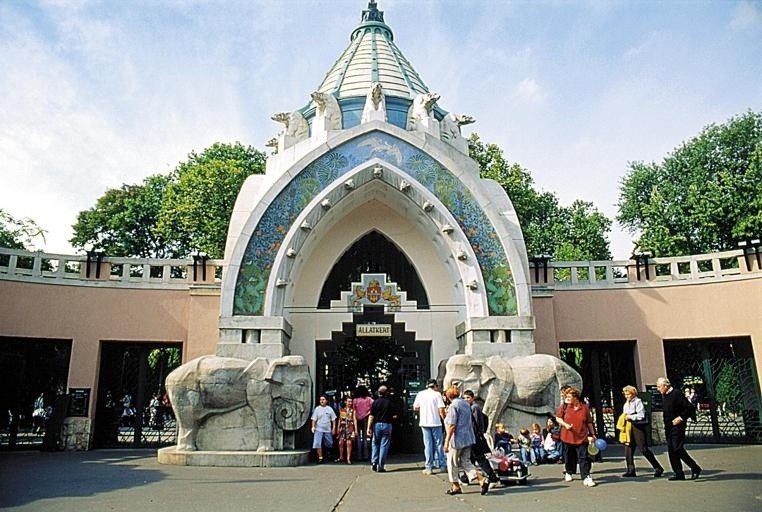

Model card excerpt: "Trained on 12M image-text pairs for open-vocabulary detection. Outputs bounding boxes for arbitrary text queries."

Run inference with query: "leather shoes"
[372,463,377,472]
[691,464,702,480]
[622,472,635,477]
[654,468,664,478]
[378,469,386,472]
[668,472,685,481]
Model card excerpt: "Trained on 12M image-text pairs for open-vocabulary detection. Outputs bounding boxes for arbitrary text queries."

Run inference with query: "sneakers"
[582,475,596,487]
[564,472,573,482]
[422,468,432,474]
[315,458,324,464]
[488,480,503,489]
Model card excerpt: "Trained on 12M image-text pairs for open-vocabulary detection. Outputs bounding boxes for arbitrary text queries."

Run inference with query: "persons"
[352,386,375,462]
[601,397,609,408]
[366,386,398,472]
[529,423,547,465]
[518,429,538,467]
[386,387,406,458]
[560,386,571,404]
[458,390,501,489]
[441,391,451,419]
[0,391,176,436]
[328,393,339,411]
[413,379,448,475]
[544,432,560,463]
[334,397,358,465]
[583,397,592,412]
[443,385,490,495]
[616,385,664,478]
[542,417,563,465]
[310,395,337,465]
[717,400,739,417]
[556,388,596,487]
[494,423,518,454]
[684,387,699,413]
[656,377,702,481]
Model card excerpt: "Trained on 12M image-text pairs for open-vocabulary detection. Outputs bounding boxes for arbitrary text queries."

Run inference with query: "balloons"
[587,445,599,455]
[595,439,606,449]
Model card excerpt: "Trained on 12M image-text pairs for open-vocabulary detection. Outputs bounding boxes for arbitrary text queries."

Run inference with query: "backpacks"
[472,409,488,434]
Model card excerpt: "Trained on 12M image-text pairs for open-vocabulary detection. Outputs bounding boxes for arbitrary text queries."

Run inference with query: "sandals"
[444,487,463,494]
[346,459,351,465]
[334,459,344,464]
[481,477,491,495]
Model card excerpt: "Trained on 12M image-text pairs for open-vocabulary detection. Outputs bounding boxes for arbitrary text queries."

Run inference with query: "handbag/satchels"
[631,398,651,428]
